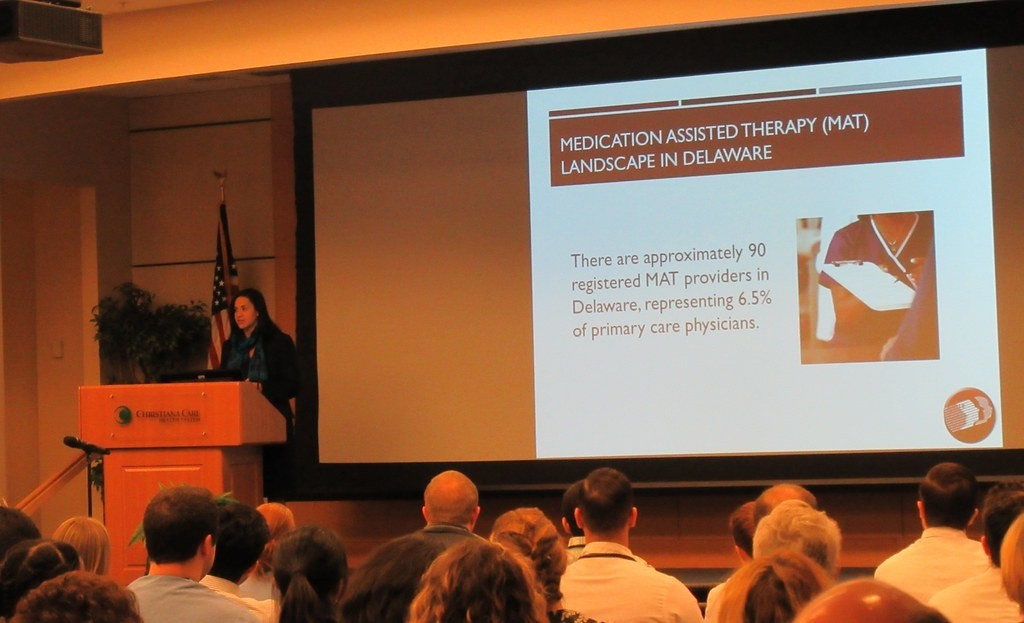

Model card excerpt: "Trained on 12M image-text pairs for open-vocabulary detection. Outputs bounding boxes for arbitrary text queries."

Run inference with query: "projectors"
[0,0,104,64]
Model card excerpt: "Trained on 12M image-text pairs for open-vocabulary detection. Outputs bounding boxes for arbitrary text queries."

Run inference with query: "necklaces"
[880,213,912,252]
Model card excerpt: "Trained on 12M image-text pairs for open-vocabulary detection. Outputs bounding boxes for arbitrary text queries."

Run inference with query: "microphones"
[64,436,110,455]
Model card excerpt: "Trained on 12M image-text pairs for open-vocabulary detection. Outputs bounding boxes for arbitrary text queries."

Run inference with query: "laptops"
[159,368,246,382]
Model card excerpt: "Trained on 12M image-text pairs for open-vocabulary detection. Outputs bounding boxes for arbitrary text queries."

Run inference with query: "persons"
[0,463,1024,623]
[818,211,939,361]
[199,287,300,503]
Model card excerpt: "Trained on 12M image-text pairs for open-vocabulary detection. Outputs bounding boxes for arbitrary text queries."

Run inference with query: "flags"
[206,201,241,370]
